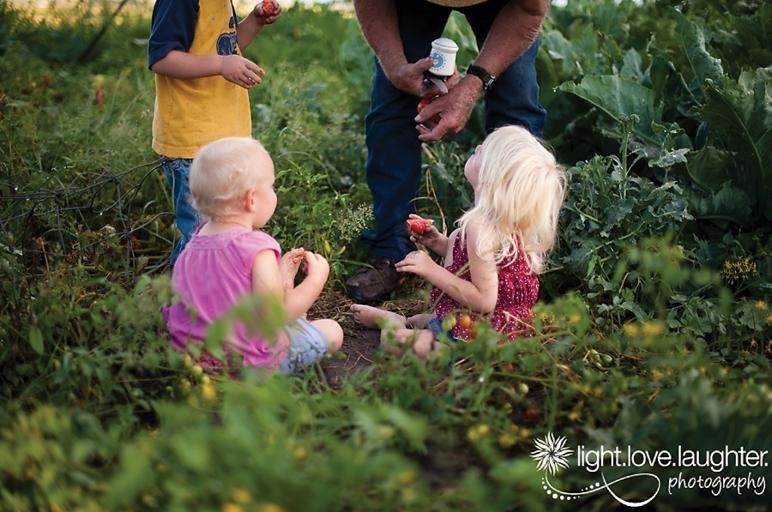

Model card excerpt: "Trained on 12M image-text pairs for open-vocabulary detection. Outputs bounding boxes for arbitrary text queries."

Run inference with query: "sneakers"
[346,249,416,305]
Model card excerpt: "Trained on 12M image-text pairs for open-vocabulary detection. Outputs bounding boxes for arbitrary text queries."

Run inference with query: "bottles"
[427,36,461,77]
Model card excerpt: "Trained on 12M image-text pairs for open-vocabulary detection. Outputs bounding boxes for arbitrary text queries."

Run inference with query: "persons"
[345,0,556,305]
[149,0,281,264]
[155,137,346,379]
[350,124,571,359]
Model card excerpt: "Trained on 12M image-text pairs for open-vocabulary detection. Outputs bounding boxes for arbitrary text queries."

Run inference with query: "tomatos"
[417,98,433,120]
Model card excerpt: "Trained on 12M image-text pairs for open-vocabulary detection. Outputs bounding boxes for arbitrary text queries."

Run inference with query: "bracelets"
[468,63,495,93]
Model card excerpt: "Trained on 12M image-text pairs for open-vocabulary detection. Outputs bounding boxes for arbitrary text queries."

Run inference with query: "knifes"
[430,78,449,94]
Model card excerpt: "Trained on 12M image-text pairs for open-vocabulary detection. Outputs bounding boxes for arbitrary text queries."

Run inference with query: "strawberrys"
[262,0,275,17]
[411,221,425,235]
